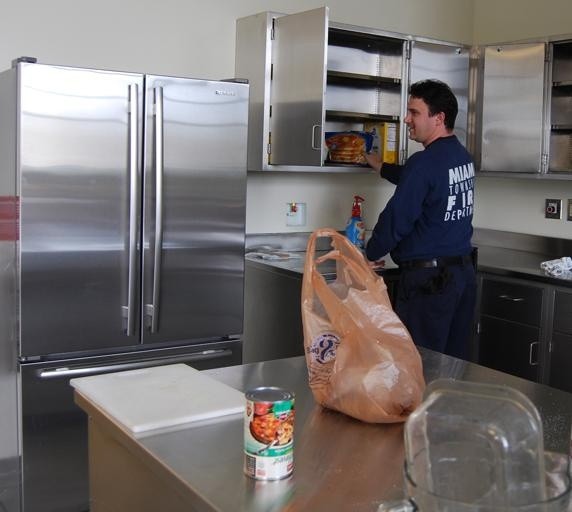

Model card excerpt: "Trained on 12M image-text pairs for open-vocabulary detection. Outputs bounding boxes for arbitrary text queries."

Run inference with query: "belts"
[396,254,474,269]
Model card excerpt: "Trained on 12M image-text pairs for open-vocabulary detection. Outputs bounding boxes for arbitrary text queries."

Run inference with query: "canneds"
[242,387,298,482]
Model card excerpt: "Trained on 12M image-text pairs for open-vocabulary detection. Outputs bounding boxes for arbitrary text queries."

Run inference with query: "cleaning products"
[345,195,366,248]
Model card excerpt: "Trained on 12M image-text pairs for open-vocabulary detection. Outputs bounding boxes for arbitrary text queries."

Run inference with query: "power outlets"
[545,199,561,220]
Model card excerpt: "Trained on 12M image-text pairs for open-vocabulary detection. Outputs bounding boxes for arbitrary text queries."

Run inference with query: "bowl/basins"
[401,457,570,511]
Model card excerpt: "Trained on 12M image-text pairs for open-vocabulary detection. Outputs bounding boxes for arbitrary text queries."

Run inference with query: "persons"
[361,79,477,359]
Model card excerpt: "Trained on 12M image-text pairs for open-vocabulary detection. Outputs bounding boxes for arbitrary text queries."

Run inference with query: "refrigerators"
[0,57,250,512]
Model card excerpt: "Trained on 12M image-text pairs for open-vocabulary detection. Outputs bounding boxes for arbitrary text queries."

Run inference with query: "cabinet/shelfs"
[545,287,572,393]
[237,6,408,172]
[406,37,471,167]
[478,275,546,381]
[481,39,572,179]
